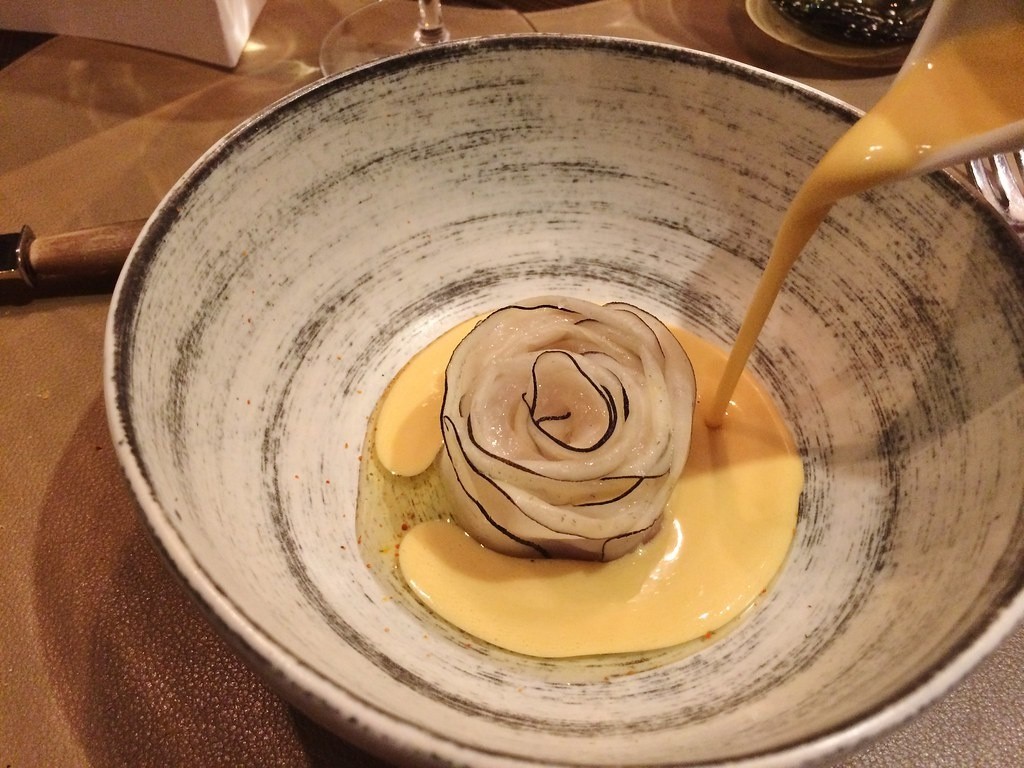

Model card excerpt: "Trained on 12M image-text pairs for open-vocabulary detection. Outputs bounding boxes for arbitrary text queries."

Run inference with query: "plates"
[746,0,921,58]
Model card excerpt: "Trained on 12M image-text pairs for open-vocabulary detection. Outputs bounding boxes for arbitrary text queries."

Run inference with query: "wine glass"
[319,0,536,79]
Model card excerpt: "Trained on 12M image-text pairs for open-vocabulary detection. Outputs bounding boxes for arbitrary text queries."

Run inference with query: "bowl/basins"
[102,33,1024,767]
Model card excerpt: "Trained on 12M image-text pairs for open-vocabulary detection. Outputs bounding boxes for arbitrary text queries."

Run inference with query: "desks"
[0,0,1024,768]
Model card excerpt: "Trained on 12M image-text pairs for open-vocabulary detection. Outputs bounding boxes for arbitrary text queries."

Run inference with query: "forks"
[966,148,1024,227]
